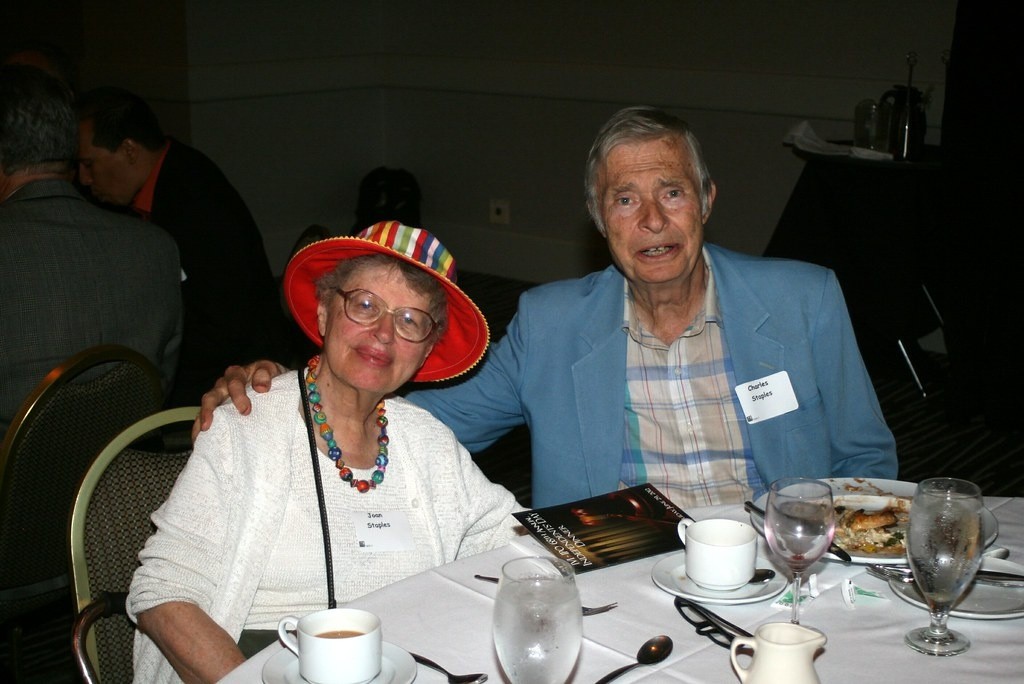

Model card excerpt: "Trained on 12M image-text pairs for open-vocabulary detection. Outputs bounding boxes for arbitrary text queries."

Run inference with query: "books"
[512,483,696,579]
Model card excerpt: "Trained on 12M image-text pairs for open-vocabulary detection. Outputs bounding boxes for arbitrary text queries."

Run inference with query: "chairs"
[0,346,165,684]
[71,407,201,684]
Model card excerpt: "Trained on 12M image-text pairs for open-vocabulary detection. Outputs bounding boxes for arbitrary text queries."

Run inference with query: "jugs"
[730,622,827,683]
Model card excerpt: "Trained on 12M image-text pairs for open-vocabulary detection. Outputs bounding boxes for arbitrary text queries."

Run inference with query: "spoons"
[594,635,674,684]
[748,569,776,584]
[410,652,488,684]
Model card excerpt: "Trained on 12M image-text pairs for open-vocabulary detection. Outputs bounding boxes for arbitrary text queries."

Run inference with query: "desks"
[216,498,1024,684]
[762,142,945,402]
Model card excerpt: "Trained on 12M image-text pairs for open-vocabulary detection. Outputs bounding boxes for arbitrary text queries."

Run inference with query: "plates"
[750,477,999,564]
[652,552,787,605]
[889,555,1014,619]
[261,642,417,684]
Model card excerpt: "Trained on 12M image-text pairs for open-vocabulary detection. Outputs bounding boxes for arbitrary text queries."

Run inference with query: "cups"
[492,556,584,684]
[277,607,381,684]
[678,518,758,591]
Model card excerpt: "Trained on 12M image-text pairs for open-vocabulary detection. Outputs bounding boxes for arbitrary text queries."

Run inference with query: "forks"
[474,574,617,615]
[868,564,1024,588]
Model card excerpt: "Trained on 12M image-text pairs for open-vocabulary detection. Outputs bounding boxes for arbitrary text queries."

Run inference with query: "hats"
[285,221,490,382]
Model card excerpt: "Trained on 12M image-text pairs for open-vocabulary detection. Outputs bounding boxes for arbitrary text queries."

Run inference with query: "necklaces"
[306,354,390,493]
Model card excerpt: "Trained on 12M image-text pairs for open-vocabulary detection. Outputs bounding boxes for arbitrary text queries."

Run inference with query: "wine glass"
[904,478,986,656]
[763,476,835,640]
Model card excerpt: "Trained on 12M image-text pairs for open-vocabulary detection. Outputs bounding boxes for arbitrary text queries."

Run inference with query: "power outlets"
[489,199,513,225]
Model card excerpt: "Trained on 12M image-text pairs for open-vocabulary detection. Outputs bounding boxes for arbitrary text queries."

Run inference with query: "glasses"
[674,596,754,649]
[333,286,440,342]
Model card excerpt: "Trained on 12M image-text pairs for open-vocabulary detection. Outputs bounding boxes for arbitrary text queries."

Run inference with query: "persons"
[126,219,533,684]
[0,62,185,603]
[192,106,900,508]
[76,85,288,434]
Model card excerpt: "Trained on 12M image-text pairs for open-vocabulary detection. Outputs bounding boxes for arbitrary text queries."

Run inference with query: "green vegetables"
[835,505,904,547]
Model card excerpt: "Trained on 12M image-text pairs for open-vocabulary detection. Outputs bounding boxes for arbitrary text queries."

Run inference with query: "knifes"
[744,500,852,563]
